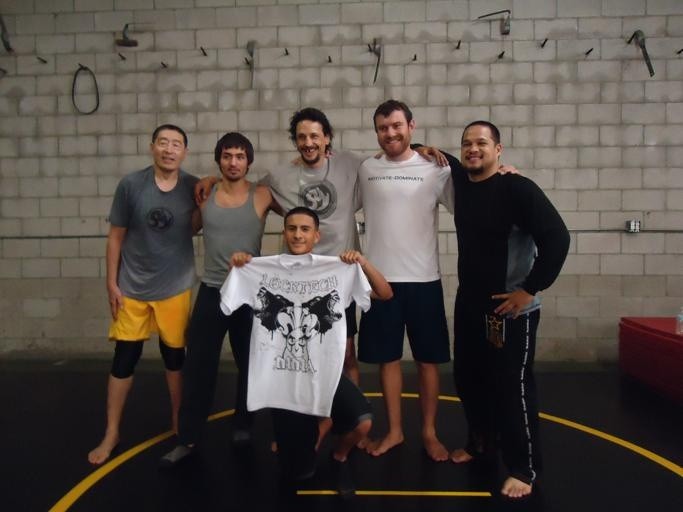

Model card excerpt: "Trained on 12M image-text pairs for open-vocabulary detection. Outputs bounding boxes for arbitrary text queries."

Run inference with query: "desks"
[618,315,683,399]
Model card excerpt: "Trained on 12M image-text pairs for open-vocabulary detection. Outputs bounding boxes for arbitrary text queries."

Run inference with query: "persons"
[193,104,451,455]
[86,122,205,469]
[161,127,275,468]
[342,100,521,463]
[407,118,570,498]
[227,206,392,471]
